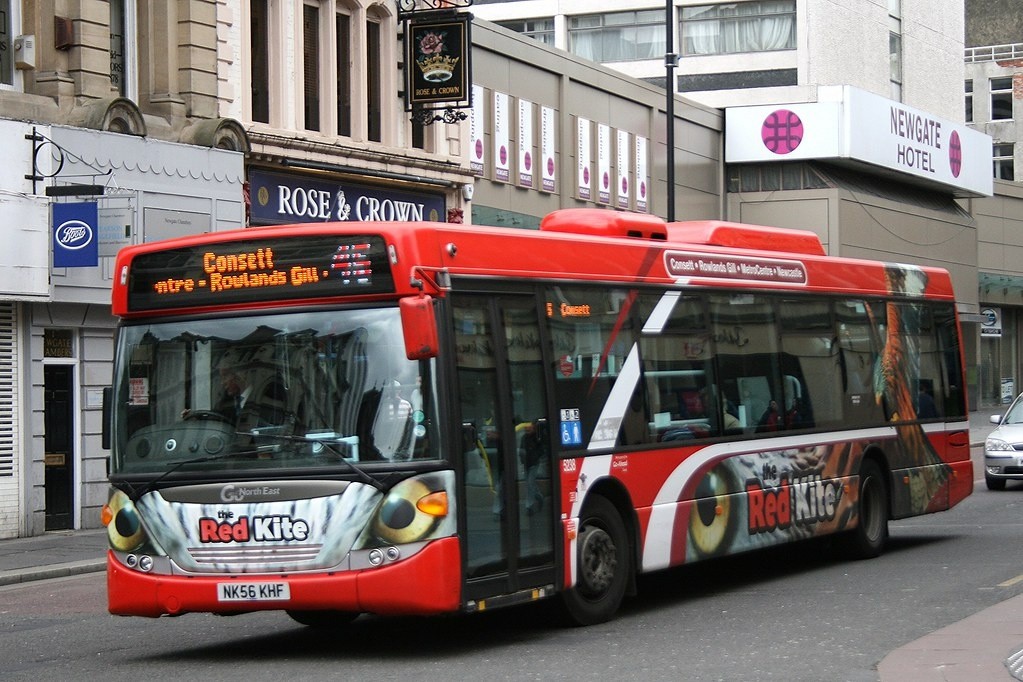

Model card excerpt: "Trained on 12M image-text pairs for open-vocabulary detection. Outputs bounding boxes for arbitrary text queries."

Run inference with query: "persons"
[180,356,268,425]
[737,361,961,433]
[666,368,742,434]
[481,423,544,520]
[381,377,425,419]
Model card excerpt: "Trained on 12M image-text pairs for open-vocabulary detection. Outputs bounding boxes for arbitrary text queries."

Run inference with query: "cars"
[984,391,1023,490]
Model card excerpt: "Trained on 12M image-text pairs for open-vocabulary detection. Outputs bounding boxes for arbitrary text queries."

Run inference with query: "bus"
[98,206,975,631]
